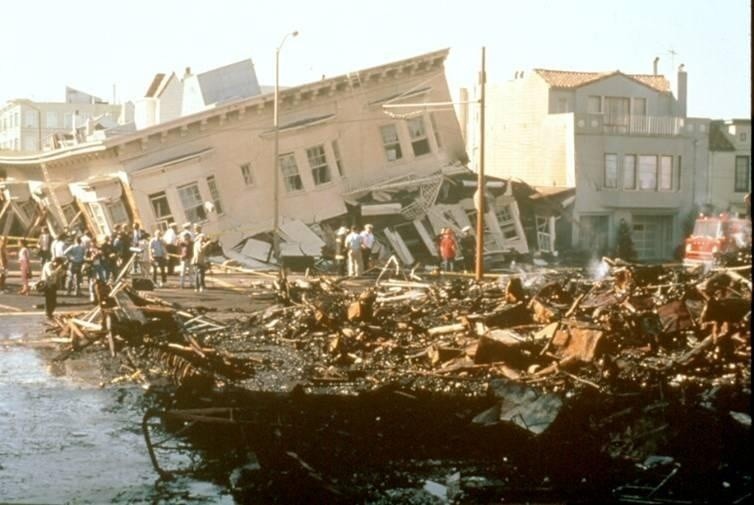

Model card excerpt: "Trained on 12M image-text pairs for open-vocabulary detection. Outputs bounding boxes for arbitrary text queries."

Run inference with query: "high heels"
[18,286,31,297]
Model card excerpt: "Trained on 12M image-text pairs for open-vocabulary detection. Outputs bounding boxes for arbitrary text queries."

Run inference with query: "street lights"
[273,28,300,253]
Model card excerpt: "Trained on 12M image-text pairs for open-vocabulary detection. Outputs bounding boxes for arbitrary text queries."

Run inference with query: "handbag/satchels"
[81,263,90,275]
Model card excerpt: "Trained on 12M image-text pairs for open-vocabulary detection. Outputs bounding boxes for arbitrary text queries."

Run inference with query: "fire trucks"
[681,211,751,268]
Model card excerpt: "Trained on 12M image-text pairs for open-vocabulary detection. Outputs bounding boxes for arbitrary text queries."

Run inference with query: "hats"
[335,224,374,236]
[462,226,470,232]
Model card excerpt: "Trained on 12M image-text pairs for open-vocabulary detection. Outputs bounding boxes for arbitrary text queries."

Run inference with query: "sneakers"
[170,220,203,239]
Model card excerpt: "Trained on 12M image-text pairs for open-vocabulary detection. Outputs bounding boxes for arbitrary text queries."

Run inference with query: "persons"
[433,226,476,274]
[0,236,10,295]
[335,223,374,280]
[17,239,32,295]
[36,221,210,320]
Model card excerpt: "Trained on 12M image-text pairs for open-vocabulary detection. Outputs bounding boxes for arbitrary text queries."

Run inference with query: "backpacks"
[31,281,47,293]
